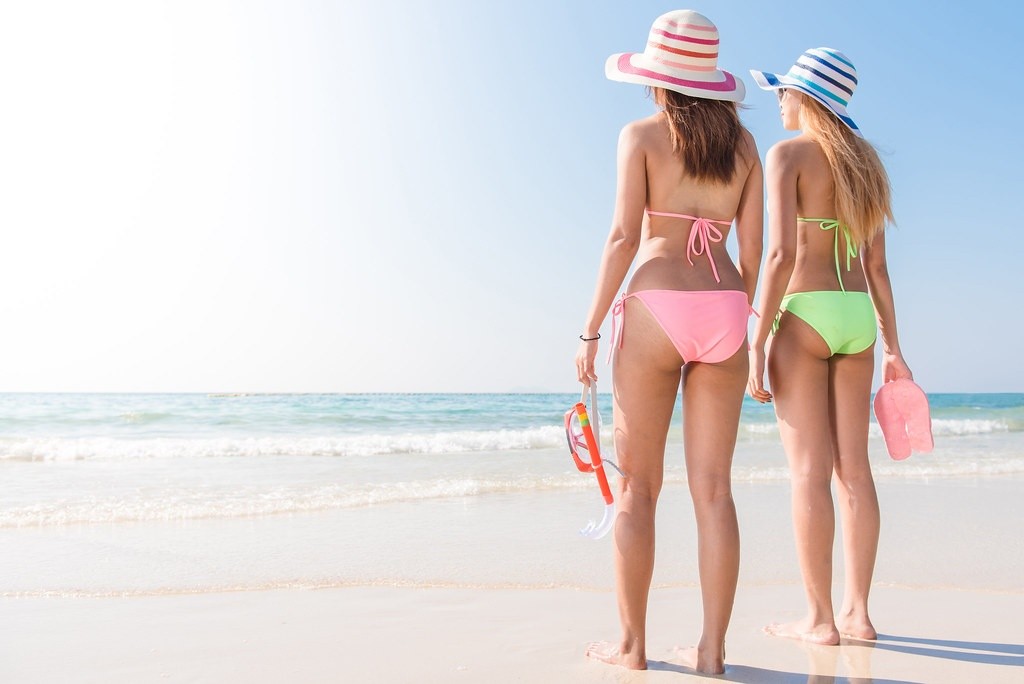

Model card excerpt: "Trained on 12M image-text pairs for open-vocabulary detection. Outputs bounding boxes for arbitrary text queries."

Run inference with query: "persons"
[575,10,916,675]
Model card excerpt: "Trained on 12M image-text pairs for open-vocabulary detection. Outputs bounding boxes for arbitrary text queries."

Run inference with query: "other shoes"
[890,379,935,455]
[873,382,912,461]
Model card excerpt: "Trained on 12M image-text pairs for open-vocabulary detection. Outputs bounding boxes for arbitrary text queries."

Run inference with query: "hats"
[604,10,746,103]
[749,46,865,140]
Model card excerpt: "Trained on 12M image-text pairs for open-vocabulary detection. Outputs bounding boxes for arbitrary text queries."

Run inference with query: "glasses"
[778,88,788,101]
[563,377,601,474]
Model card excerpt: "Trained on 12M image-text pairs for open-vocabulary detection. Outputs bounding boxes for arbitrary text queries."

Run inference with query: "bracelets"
[579,333,601,341]
